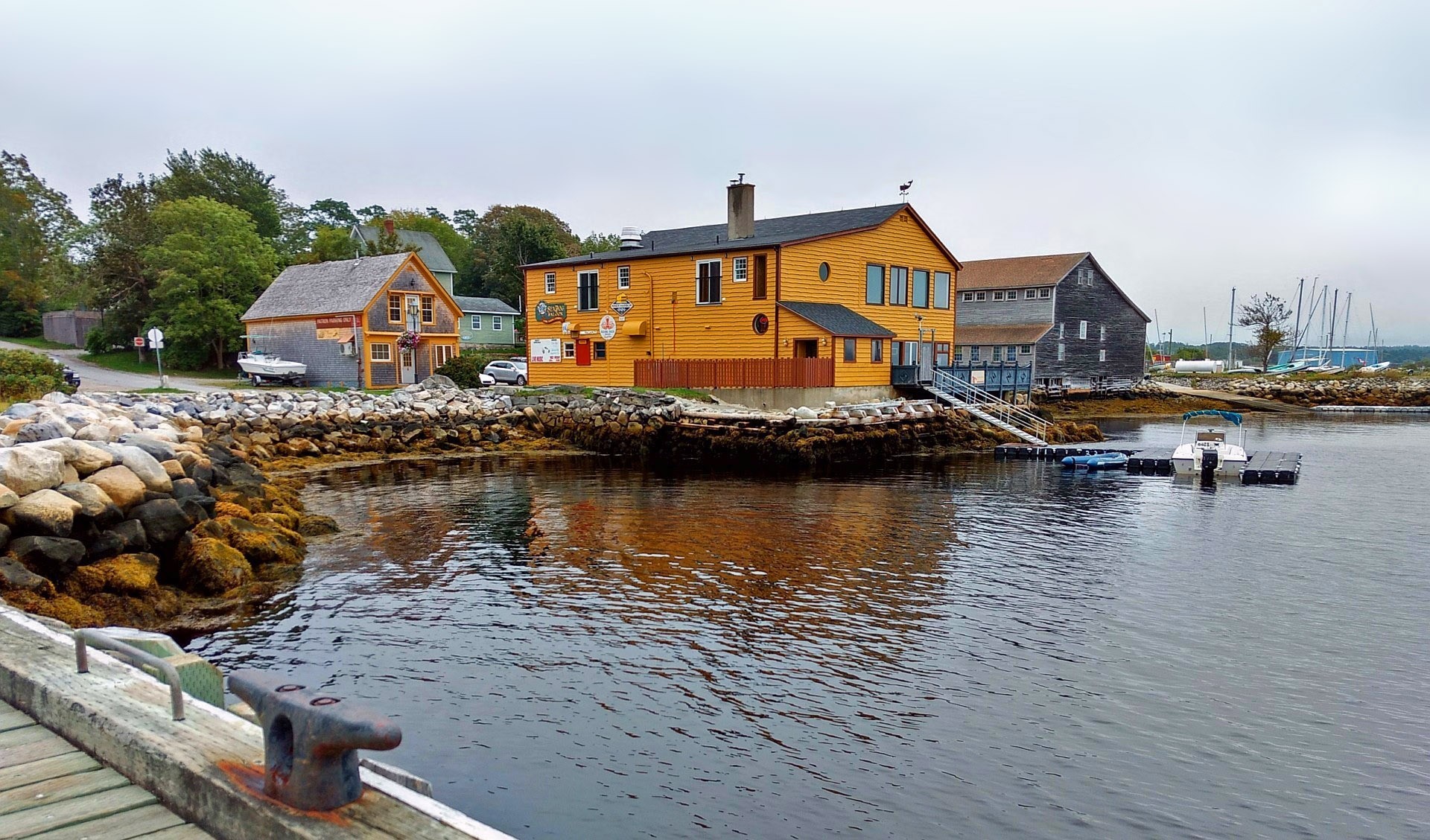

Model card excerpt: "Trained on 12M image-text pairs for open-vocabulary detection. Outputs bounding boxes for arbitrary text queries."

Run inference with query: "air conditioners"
[339,342,352,356]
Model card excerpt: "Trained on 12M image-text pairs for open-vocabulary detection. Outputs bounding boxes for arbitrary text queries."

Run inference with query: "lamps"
[822,336,827,346]
[783,337,788,347]
[411,278,415,285]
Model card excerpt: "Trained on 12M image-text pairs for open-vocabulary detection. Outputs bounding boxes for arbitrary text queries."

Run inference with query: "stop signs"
[134,337,145,347]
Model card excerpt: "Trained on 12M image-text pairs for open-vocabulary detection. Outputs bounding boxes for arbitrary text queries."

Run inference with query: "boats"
[1172,408,1249,477]
[236,335,307,376]
[1062,452,1128,469]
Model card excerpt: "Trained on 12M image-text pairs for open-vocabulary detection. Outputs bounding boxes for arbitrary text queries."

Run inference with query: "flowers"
[395,329,421,355]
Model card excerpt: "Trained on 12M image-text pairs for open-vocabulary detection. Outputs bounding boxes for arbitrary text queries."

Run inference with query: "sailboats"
[1148,309,1166,373]
[1358,301,1390,373]
[1223,275,1351,375]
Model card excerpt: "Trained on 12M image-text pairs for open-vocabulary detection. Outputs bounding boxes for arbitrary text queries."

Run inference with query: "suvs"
[483,360,527,385]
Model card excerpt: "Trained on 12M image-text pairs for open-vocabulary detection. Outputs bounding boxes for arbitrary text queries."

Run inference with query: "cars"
[479,373,494,387]
[61,366,80,388]
